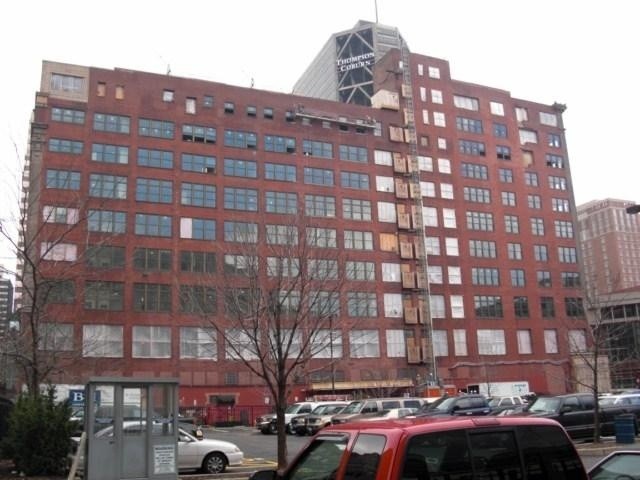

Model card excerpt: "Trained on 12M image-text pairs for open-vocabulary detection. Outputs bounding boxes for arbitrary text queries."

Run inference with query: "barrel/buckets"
[614,414,635,443]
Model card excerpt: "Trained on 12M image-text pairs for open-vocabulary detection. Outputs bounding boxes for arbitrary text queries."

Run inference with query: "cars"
[66,405,244,480]
